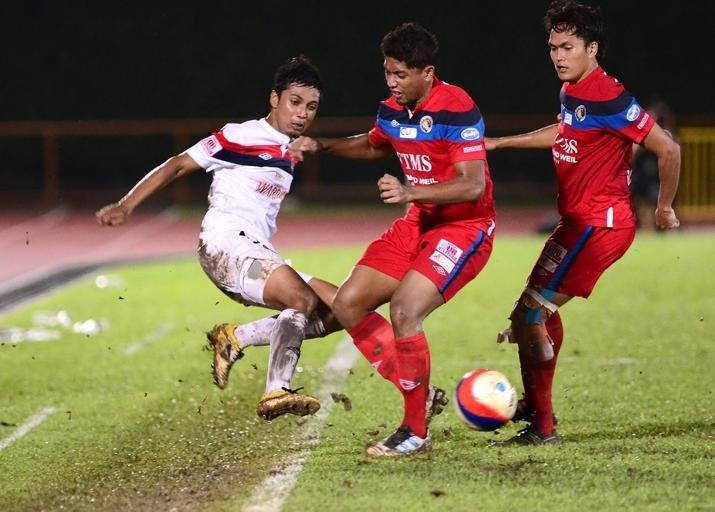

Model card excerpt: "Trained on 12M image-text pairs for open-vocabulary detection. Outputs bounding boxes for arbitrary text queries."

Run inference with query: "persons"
[94,56,345,422]
[287,22,498,457]
[633,101,680,229]
[484,4,682,448]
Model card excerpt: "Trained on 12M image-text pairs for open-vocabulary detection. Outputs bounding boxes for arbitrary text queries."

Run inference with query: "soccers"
[454,369,518,432]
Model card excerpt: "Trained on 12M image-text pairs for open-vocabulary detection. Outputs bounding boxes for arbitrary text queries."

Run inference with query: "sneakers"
[491,426,562,447]
[257,388,321,421]
[212,322,245,391]
[423,383,449,425]
[364,424,431,460]
[511,398,559,426]
